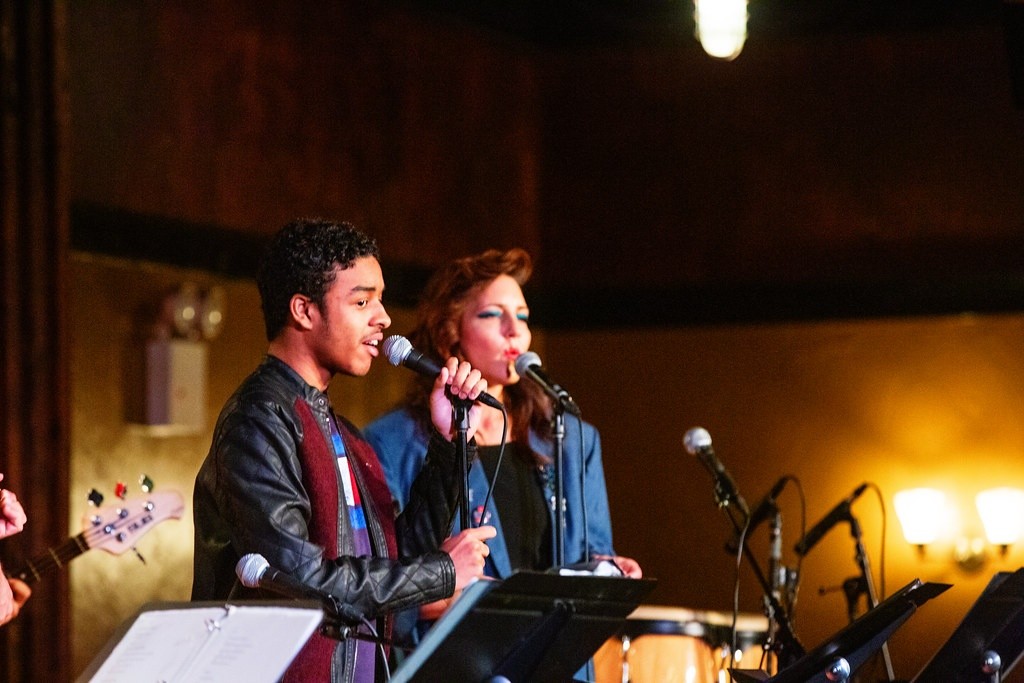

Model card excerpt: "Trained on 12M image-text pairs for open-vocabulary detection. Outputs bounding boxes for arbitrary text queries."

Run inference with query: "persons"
[189,218,498,683]
[0,472,31,627]
[360,248,644,683]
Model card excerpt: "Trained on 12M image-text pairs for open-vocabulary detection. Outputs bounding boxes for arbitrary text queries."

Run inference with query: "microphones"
[724,476,791,555]
[795,483,868,557]
[235,554,365,625]
[513,351,582,417]
[682,426,748,516]
[381,334,505,410]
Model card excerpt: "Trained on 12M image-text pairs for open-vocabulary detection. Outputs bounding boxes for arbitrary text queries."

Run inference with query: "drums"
[591,605,779,683]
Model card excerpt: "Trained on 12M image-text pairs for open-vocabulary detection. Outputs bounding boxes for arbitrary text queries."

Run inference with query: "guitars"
[7,475,185,591]
